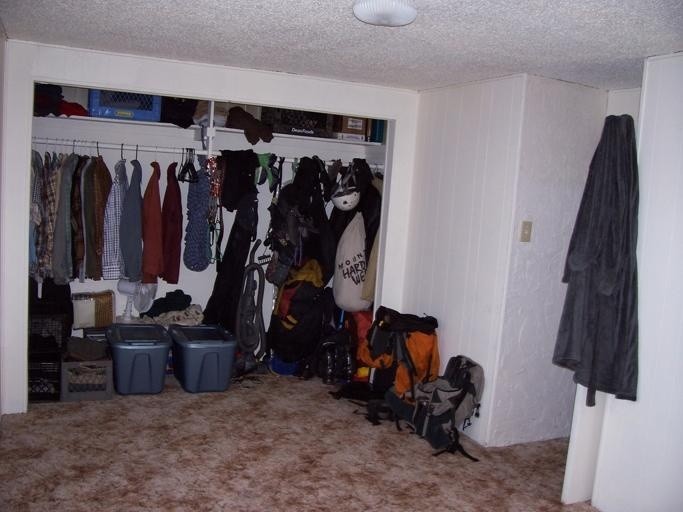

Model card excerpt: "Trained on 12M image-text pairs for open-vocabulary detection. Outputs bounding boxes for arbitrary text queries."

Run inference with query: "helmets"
[330,186,361,211]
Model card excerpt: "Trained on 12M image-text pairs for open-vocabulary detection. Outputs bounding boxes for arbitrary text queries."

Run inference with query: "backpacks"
[385,325,440,431]
[410,355,484,463]
[357,306,405,398]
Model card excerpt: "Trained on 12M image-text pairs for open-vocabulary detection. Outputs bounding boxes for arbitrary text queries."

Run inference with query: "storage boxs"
[29,348,61,403]
[89,90,163,122]
[105,323,172,395]
[170,323,238,393]
[332,116,366,135]
[61,337,114,401]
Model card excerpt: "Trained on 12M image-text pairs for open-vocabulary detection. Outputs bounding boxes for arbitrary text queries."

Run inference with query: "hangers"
[176,147,200,184]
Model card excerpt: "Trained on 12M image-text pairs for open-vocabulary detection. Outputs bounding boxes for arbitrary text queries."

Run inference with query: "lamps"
[353,0,417,27]
